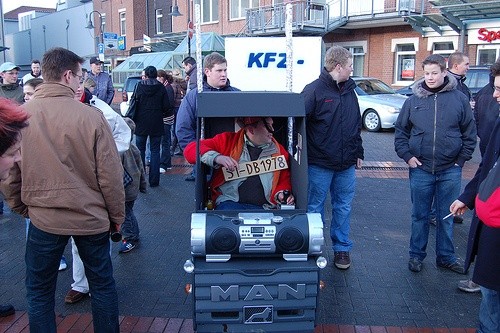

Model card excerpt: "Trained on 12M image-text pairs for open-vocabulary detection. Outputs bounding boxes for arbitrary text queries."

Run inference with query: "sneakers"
[408,257,423,271]
[334,251,352,269]
[436,259,465,274]
[457,279,481,291]
[118,240,136,252]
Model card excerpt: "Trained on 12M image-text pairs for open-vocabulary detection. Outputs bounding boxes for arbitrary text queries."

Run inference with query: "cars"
[348,76,411,132]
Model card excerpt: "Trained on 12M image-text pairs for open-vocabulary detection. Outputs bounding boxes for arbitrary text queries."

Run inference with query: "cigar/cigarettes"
[442,212,453,220]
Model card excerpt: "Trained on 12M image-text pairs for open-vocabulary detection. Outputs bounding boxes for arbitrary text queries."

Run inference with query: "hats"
[236,117,267,128]
[90,57,104,65]
[0,62,21,73]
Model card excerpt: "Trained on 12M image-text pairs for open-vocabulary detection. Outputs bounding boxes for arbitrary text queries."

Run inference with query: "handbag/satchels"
[125,82,139,119]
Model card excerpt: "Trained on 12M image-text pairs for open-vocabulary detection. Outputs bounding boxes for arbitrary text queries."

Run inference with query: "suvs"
[396,64,491,113]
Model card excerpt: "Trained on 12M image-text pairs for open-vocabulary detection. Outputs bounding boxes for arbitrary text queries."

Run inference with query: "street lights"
[85,10,105,73]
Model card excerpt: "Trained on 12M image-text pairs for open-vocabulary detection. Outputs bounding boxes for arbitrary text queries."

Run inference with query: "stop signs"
[188,20,194,38]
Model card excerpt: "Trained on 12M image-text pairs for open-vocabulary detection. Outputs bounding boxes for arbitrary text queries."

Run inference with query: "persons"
[183,117,295,211]
[430,52,500,333]
[0,52,245,303]
[300,46,365,269]
[0,95,32,316]
[0,47,126,333]
[394,54,477,274]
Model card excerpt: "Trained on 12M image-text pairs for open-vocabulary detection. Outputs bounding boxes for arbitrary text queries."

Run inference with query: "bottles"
[110,223,122,242]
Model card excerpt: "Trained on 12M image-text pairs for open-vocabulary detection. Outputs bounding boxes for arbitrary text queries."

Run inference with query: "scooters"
[183,86,328,333]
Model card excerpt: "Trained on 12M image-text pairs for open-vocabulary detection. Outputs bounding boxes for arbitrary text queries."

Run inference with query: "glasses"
[63,71,83,83]
[492,85,500,95]
[3,71,19,76]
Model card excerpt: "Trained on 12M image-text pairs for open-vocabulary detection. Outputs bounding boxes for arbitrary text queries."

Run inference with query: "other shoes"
[170,151,174,155]
[430,217,437,226]
[185,176,195,181]
[58,254,67,270]
[144,166,151,176]
[453,216,463,224]
[0,304,15,318]
[149,182,159,187]
[65,289,86,303]
[160,168,166,174]
[174,148,182,155]
